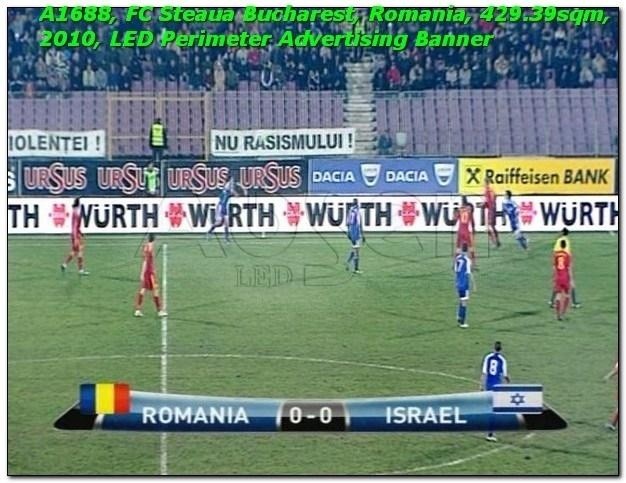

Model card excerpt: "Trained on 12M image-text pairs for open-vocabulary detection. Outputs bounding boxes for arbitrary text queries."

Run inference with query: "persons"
[131,231,168,318]
[344,199,366,274]
[148,115,170,161]
[552,239,573,319]
[452,241,477,328]
[7,7,618,98]
[143,164,159,197]
[549,226,582,309]
[501,190,529,252]
[477,178,501,249]
[453,195,476,259]
[600,361,619,430]
[60,195,89,275]
[206,175,236,244]
[478,340,511,442]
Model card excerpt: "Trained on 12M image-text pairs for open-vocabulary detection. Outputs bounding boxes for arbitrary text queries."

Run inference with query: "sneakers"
[606,422,616,432]
[344,261,364,273]
[206,230,231,246]
[547,300,583,321]
[486,436,497,441]
[61,263,90,275]
[460,324,468,329]
[133,309,168,318]
